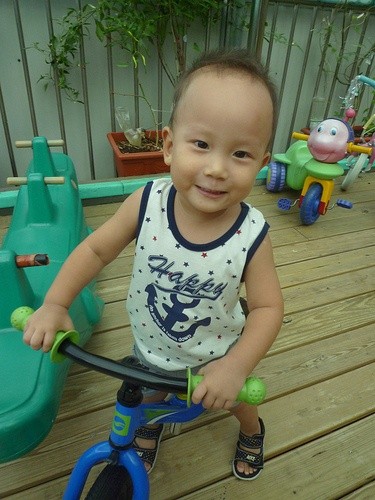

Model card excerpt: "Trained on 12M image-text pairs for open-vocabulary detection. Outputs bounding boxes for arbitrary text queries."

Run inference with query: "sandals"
[232,416,265,482]
[131,420,166,475]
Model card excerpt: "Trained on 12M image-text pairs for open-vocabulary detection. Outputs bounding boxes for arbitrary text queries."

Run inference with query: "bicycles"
[9,305,267,500]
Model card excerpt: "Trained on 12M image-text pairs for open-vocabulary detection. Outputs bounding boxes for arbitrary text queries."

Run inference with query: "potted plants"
[22,0,375,178]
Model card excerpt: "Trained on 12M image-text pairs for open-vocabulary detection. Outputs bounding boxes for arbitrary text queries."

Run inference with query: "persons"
[23,44,284,482]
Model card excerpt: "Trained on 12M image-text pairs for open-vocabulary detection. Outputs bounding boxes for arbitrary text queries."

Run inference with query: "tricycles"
[263,116,374,226]
